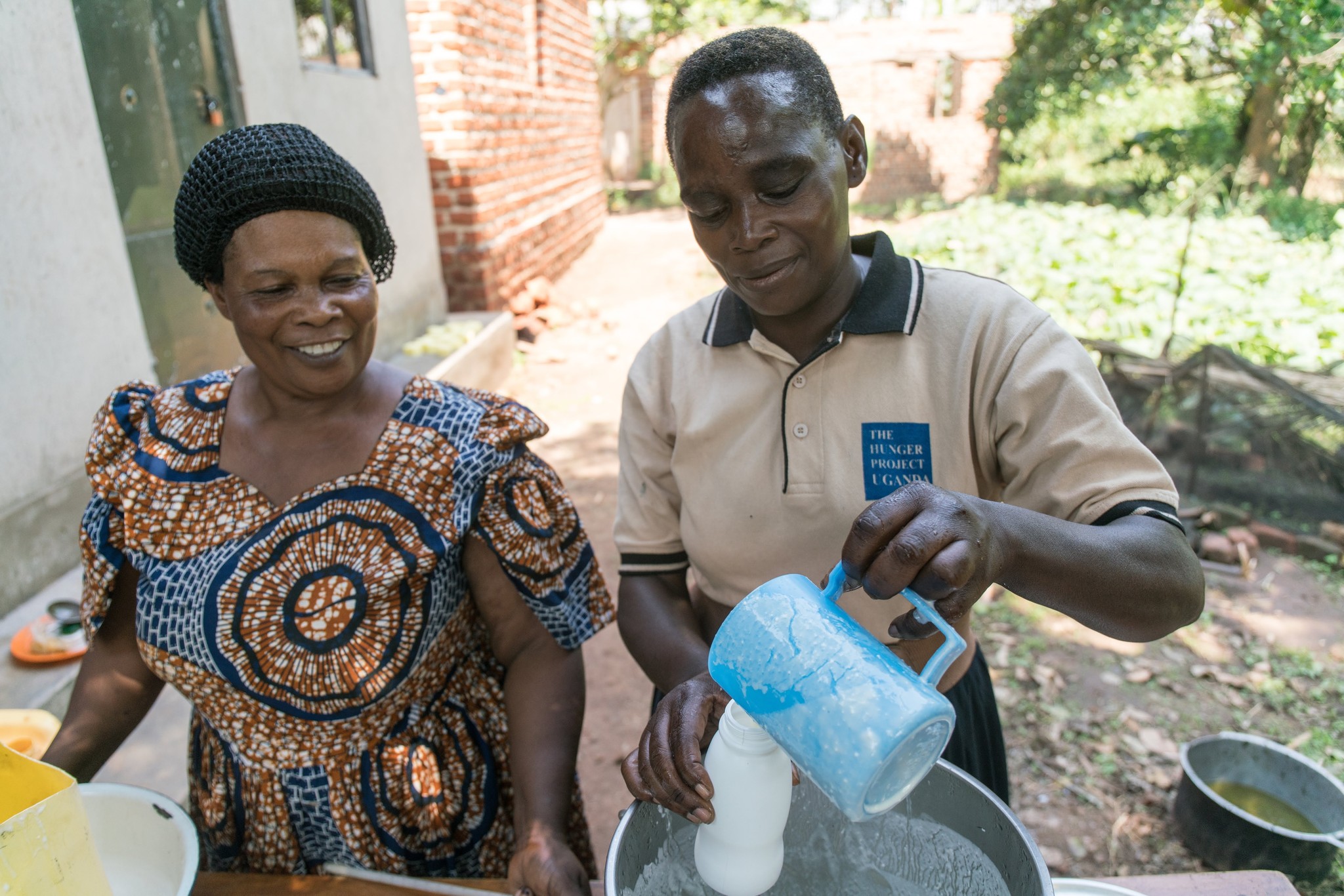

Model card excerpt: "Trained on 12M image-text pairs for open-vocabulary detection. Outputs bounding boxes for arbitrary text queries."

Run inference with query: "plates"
[10,613,89,663]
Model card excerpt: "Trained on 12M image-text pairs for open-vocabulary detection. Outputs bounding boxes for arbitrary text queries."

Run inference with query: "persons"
[609,29,1211,827]
[33,121,617,896]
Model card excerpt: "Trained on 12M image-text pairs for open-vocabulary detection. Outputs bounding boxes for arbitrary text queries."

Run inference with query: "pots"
[1174,732,1343,890]
[604,757,1054,896]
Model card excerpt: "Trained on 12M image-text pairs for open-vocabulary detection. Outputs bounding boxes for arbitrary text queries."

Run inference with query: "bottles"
[694,699,791,896]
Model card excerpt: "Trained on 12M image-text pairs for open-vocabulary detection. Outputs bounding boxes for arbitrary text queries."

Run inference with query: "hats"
[171,121,395,291]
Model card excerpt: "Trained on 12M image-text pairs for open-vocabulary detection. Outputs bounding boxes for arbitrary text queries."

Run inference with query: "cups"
[708,557,967,821]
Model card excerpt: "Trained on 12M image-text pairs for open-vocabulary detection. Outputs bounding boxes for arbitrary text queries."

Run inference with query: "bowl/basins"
[79,784,199,896]
